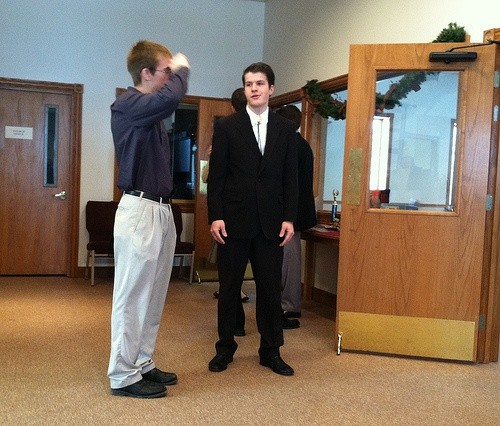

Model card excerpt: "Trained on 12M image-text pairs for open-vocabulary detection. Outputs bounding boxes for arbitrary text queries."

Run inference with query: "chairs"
[169,203,196,286]
[85,201,120,287]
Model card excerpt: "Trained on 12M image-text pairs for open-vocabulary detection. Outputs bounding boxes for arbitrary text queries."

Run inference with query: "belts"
[125,190,174,205]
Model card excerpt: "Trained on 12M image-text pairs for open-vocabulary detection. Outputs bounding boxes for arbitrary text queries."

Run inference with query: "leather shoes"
[209,353,233,372]
[110,380,168,398]
[142,368,177,385]
[260,357,294,375]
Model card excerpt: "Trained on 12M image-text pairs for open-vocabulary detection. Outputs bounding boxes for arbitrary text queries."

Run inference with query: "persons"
[106,40,190,399]
[206,62,316,375]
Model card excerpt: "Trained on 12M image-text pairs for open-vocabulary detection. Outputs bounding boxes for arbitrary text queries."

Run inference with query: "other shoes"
[283,310,301,318]
[283,317,301,329]
[236,328,246,336]
[240,296,249,301]
[212,290,220,296]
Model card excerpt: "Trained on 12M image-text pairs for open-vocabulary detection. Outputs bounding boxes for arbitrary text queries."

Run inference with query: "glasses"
[156,69,174,79]
